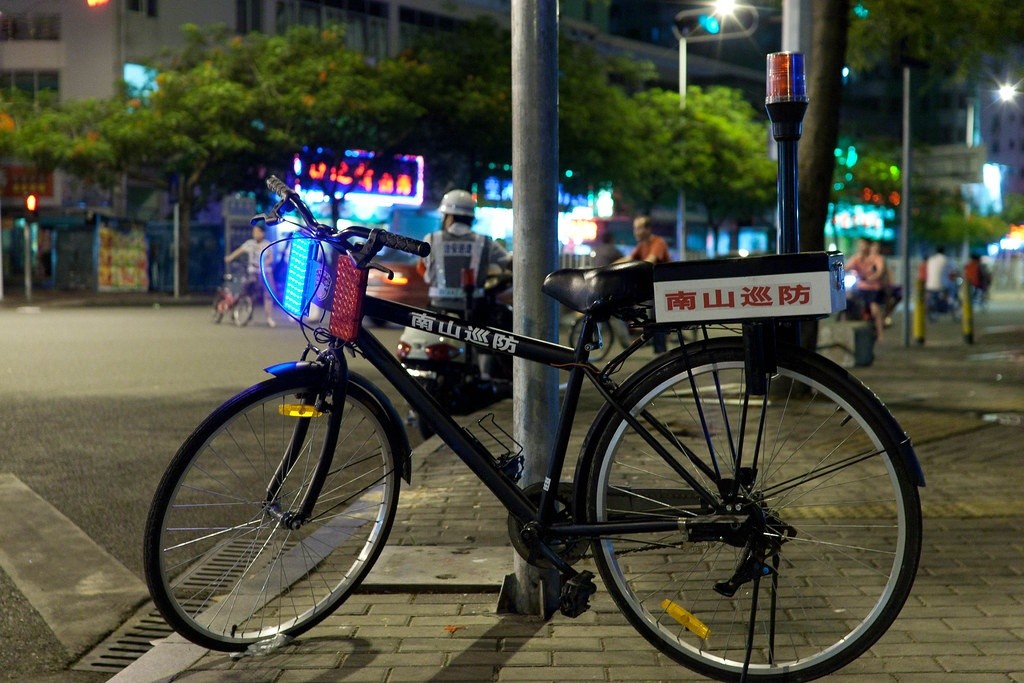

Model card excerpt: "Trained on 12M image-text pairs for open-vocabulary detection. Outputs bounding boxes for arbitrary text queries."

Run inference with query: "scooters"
[399,271,513,441]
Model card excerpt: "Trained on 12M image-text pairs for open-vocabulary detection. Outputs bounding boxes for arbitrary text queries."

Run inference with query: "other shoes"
[266,317,277,328]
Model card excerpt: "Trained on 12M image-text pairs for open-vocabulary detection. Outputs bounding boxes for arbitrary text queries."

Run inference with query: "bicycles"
[213,263,264,327]
[568,303,698,362]
[144,51,925,683]
[928,280,986,322]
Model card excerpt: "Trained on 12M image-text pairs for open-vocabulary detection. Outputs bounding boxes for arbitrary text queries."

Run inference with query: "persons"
[612,215,672,355]
[590,232,624,268]
[924,245,957,310]
[224,226,276,327]
[420,190,513,380]
[918,256,926,281]
[964,253,987,291]
[845,237,901,341]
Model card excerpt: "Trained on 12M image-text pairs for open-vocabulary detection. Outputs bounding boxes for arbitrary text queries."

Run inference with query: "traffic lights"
[25,194,39,222]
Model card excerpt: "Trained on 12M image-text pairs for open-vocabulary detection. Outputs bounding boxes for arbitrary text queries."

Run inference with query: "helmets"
[438,190,476,217]
